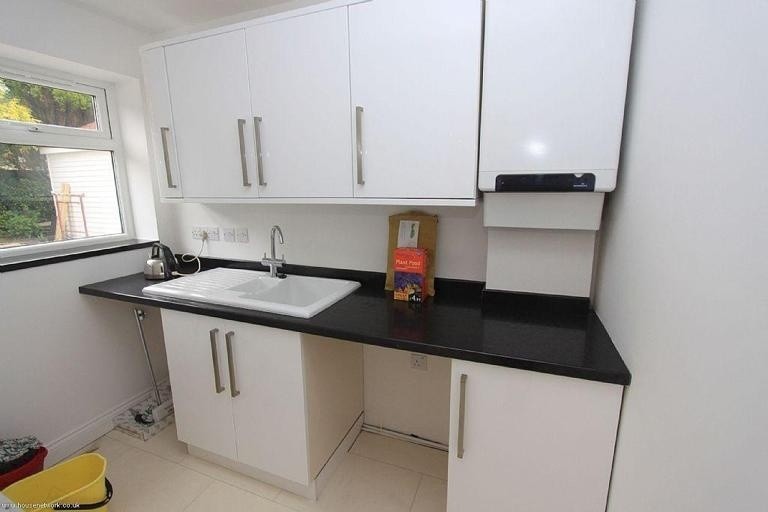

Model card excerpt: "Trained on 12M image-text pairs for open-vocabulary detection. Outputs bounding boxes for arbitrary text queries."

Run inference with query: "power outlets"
[192,226,219,240]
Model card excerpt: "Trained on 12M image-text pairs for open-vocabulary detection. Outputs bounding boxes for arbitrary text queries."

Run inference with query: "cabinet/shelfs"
[136,40,184,205]
[162,1,352,206]
[348,1,484,207]
[160,308,363,499]
[446,357,624,512]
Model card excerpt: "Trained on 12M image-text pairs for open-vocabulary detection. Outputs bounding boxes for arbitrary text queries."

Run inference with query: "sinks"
[223,273,362,319]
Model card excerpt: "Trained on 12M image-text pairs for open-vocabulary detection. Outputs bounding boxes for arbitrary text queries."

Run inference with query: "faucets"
[260,225,284,277]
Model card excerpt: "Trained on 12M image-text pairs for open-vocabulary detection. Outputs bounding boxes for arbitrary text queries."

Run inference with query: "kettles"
[144,242,180,281]
